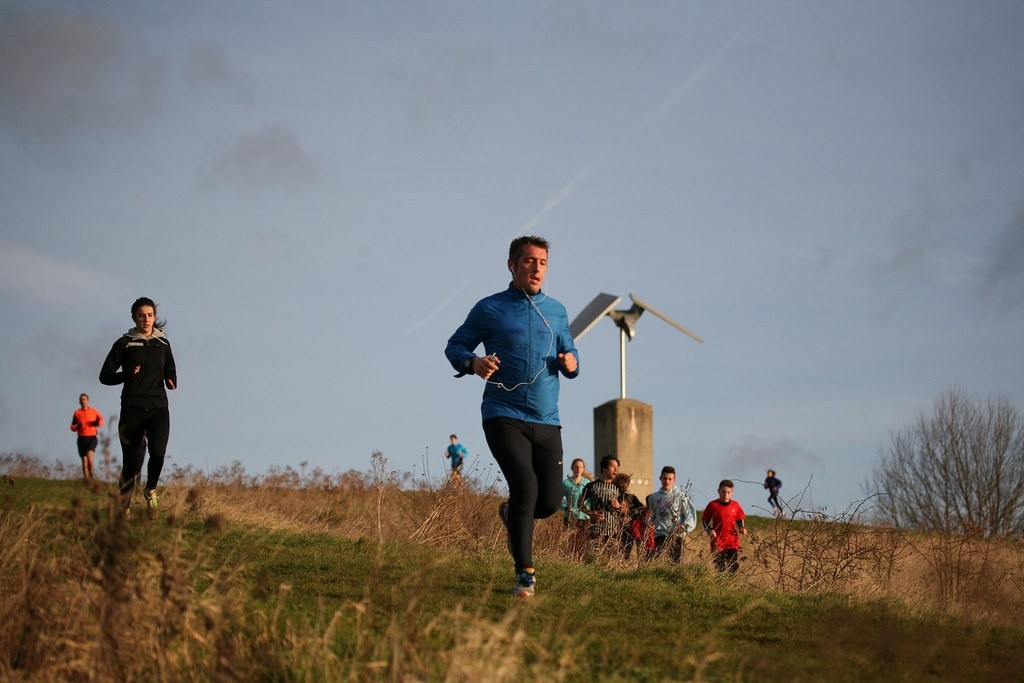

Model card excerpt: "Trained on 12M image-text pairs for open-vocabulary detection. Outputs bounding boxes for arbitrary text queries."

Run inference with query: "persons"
[578,455,625,543]
[99,297,177,525]
[444,236,580,598]
[562,458,593,532]
[701,480,748,574]
[763,469,786,520]
[444,433,468,478]
[616,466,698,564]
[70,393,103,482]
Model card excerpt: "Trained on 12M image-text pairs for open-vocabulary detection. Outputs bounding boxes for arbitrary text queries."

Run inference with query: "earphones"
[510,265,514,273]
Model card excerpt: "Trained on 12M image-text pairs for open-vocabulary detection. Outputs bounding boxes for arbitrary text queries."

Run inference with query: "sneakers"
[498,502,513,557]
[123,507,131,520]
[512,569,536,597]
[142,488,158,520]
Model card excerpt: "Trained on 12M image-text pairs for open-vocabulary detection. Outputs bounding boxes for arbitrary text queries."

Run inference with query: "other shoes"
[774,508,779,516]
[780,512,785,521]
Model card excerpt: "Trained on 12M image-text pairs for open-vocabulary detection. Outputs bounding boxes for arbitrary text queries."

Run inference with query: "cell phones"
[490,352,497,363]
